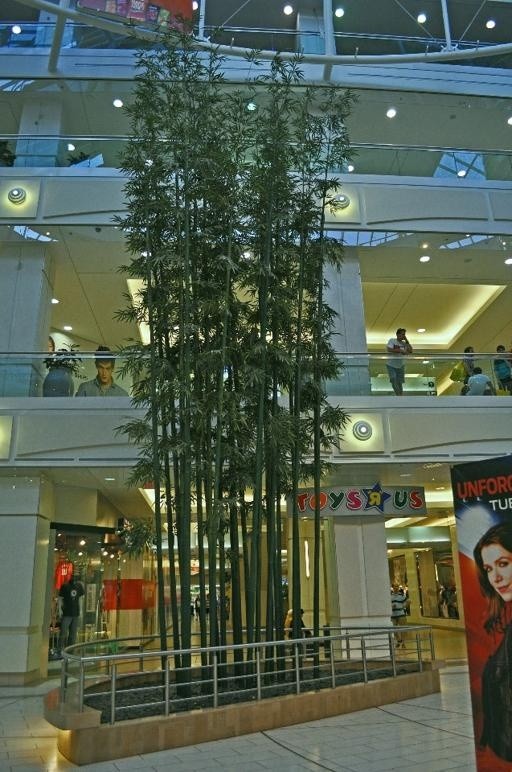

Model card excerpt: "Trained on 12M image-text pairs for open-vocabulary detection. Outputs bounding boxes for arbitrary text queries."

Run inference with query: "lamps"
[9,188,27,205]
[333,194,350,210]
[351,419,374,445]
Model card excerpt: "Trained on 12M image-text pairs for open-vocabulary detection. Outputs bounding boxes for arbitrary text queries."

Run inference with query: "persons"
[492,344,511,394]
[57,576,86,652]
[205,593,212,620]
[194,594,201,621]
[74,344,130,397]
[461,347,475,383]
[471,517,511,768]
[47,336,57,352]
[460,367,497,396]
[434,577,459,618]
[384,328,413,395]
[288,608,312,679]
[391,583,413,649]
[215,594,221,618]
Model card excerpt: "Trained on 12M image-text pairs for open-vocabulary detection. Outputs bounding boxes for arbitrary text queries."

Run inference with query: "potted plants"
[41,343,83,396]
